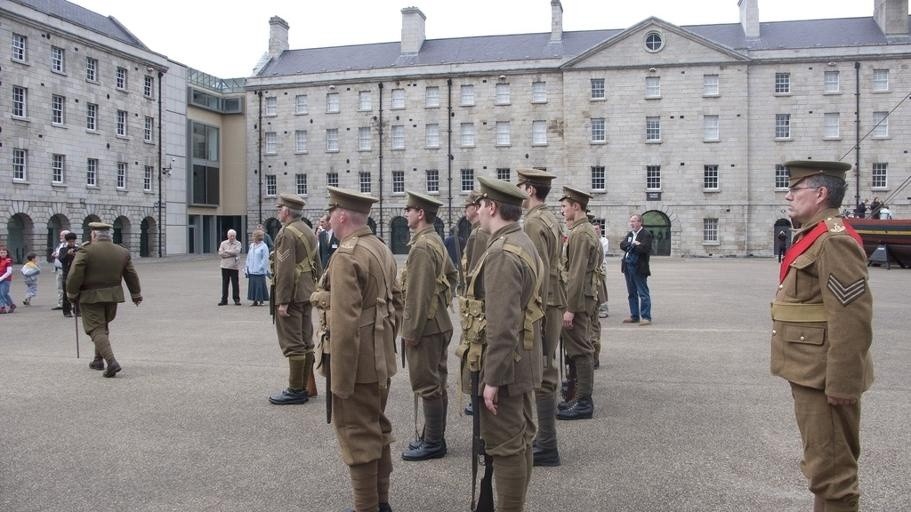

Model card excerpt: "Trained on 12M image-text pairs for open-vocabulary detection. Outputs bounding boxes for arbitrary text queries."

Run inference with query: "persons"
[314,182,401,512]
[50,226,70,313]
[256,223,275,280]
[0,245,17,315]
[856,195,893,221]
[776,229,788,262]
[57,233,84,319]
[63,221,145,379]
[216,226,244,307]
[309,169,609,466]
[21,251,41,306]
[618,212,652,327]
[457,173,546,512]
[243,230,270,308]
[769,158,874,512]
[266,191,317,407]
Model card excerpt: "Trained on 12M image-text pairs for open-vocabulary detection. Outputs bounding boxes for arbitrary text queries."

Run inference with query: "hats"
[599,304,608,317]
[515,168,557,186]
[275,193,306,210]
[88,222,113,231]
[404,191,443,213]
[461,190,481,205]
[324,186,379,213]
[559,185,593,205]
[476,177,528,206]
[782,161,852,188]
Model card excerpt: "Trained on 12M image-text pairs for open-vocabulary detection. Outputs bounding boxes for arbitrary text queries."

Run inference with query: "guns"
[324,330,333,420]
[468,356,497,512]
[72,302,83,359]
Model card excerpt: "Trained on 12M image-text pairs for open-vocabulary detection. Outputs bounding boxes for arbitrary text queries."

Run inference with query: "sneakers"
[622,317,639,323]
[0,307,6,314]
[89,360,104,370]
[64,312,72,317]
[74,312,81,316]
[235,302,241,305]
[104,361,121,376]
[638,319,651,325]
[218,302,228,305]
[8,304,16,313]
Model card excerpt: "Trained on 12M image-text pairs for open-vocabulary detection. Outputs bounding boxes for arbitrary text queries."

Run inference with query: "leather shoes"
[268,389,307,404]
[343,397,594,512]
